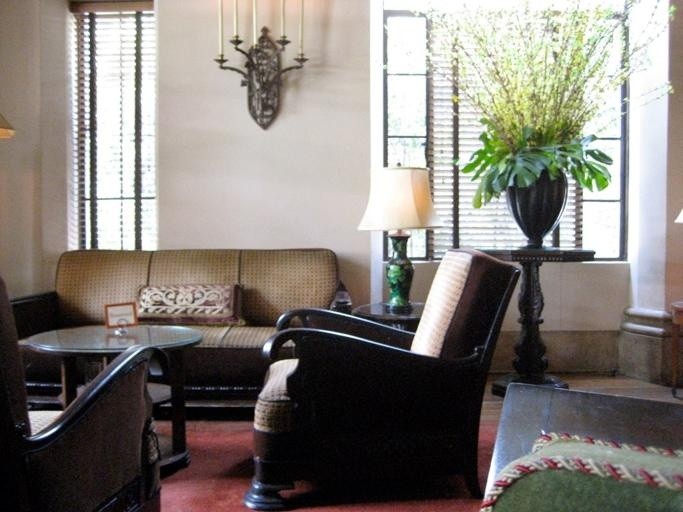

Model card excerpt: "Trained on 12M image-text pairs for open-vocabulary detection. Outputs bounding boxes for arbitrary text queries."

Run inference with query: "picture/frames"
[102,300,139,329]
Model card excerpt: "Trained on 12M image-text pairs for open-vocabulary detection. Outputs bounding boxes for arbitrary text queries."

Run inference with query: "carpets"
[151,418,498,511]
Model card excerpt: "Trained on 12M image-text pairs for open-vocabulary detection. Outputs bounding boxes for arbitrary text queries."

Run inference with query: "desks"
[16,323,205,483]
[490,246,597,399]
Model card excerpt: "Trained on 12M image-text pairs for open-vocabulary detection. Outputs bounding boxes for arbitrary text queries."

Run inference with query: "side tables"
[348,300,427,334]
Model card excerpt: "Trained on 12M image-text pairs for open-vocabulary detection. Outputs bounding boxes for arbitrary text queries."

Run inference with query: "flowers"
[407,0,679,201]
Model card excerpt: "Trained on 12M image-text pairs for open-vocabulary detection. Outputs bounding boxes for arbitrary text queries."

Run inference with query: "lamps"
[354,167,442,314]
[213,1,309,131]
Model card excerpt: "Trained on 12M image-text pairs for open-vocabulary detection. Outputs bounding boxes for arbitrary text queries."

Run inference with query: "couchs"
[0,282,164,512]
[8,247,357,422]
[242,245,520,512]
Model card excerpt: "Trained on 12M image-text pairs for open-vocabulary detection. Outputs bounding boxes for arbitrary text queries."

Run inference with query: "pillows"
[133,284,244,326]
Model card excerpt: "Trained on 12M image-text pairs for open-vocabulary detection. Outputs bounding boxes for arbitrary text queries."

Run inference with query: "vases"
[496,173,571,254]
